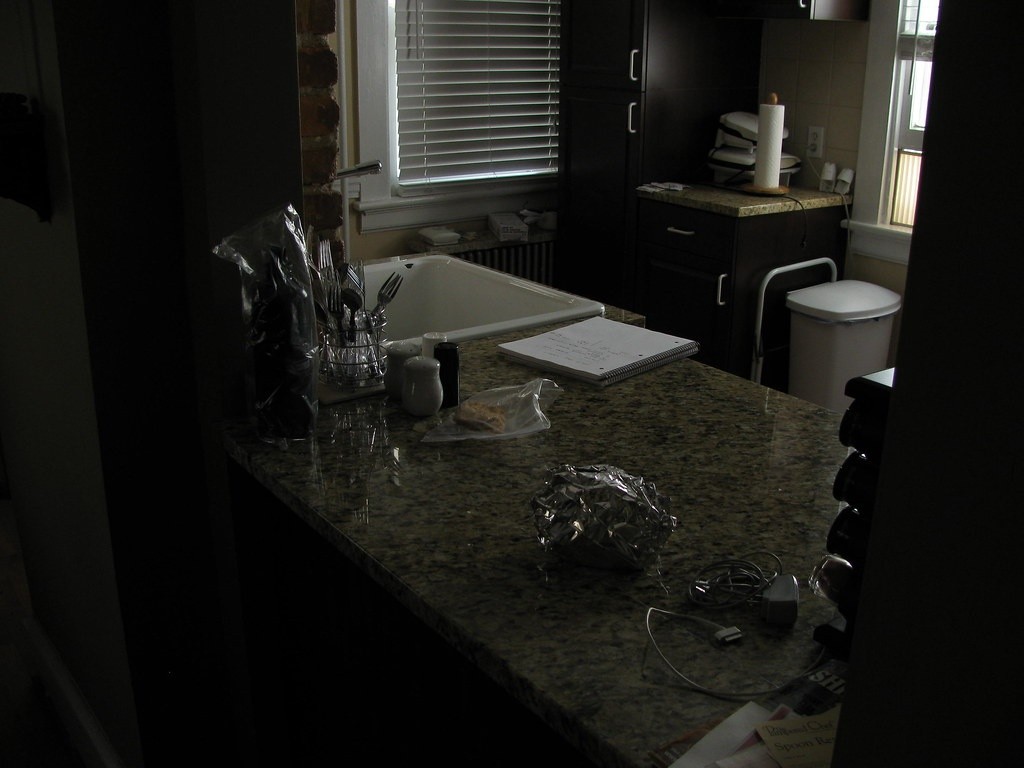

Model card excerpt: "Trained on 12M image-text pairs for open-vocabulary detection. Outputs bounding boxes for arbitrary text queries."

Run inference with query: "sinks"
[361,253,606,357]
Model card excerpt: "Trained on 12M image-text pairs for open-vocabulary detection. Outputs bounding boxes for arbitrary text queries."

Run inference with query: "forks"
[372,271,404,314]
[348,268,365,312]
[329,286,346,343]
[318,239,335,278]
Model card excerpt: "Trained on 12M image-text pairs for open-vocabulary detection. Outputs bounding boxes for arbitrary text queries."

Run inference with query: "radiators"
[450,240,555,286]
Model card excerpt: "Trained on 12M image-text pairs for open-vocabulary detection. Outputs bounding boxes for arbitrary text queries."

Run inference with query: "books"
[496,315,699,387]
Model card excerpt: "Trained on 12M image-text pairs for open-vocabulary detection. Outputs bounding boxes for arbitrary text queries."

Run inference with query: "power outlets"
[807,126,824,158]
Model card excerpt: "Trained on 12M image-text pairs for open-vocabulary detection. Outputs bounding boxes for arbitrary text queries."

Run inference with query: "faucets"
[335,160,383,181]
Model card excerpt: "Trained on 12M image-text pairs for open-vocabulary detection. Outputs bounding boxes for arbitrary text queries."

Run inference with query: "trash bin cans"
[785,279,902,414]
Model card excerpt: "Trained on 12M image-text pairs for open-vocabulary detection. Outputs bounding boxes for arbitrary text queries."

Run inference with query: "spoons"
[348,289,363,343]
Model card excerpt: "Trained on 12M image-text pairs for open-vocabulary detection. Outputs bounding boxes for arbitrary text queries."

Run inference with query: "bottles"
[434,343,460,405]
[422,332,447,355]
[403,355,443,417]
[384,340,421,400]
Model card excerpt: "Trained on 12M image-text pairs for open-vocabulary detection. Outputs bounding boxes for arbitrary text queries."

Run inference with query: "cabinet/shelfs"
[560,0,870,389]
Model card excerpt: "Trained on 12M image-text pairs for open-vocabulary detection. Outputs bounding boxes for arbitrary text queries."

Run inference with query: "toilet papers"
[753,104,785,188]
